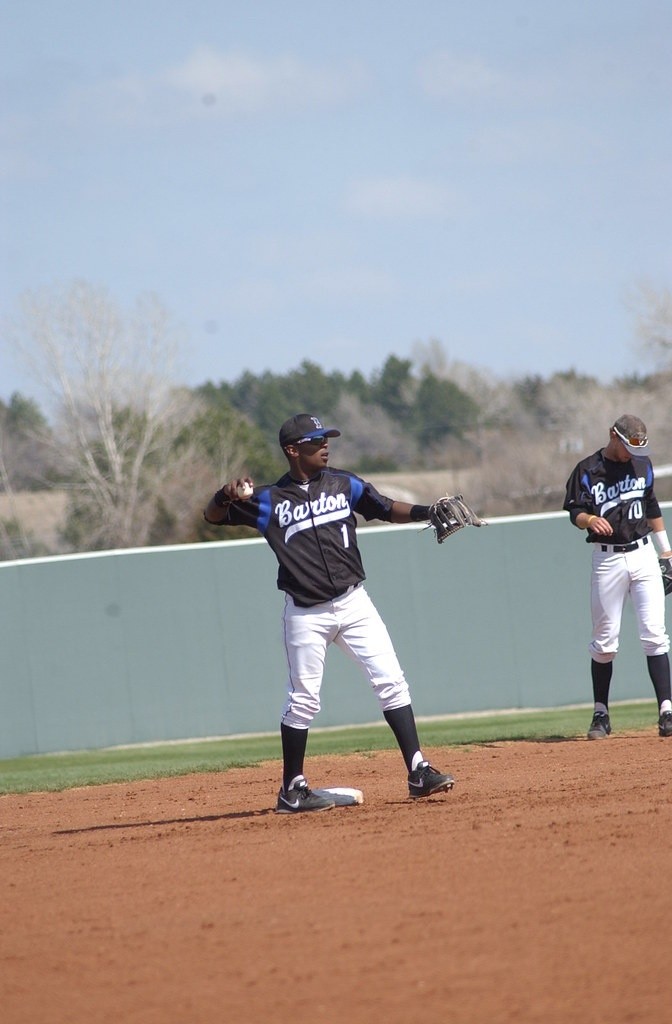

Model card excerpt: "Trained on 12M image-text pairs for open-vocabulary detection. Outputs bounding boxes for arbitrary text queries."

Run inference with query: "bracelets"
[588,516,596,525]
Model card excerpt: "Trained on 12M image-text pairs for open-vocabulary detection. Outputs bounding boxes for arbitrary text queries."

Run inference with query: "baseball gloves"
[658,555,672,597]
[429,495,483,544]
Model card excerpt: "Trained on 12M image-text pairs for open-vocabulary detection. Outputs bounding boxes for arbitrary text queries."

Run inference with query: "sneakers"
[407,760,455,800]
[274,778,335,813]
[587,711,611,741]
[658,710,672,737]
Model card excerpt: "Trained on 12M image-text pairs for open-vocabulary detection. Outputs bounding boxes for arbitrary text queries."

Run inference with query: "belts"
[332,582,358,598]
[602,537,648,553]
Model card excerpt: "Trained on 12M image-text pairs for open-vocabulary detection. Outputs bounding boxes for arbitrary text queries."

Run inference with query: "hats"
[613,415,650,457]
[279,414,340,449]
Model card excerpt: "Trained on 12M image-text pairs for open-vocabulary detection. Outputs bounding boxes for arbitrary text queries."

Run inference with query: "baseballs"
[236,482,253,499]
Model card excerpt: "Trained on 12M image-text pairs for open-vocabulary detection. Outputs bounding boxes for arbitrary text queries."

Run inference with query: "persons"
[562,414,672,738]
[203,415,482,814]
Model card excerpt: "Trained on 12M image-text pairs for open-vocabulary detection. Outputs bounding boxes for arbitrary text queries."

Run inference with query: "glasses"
[614,426,648,448]
[291,435,329,446]
